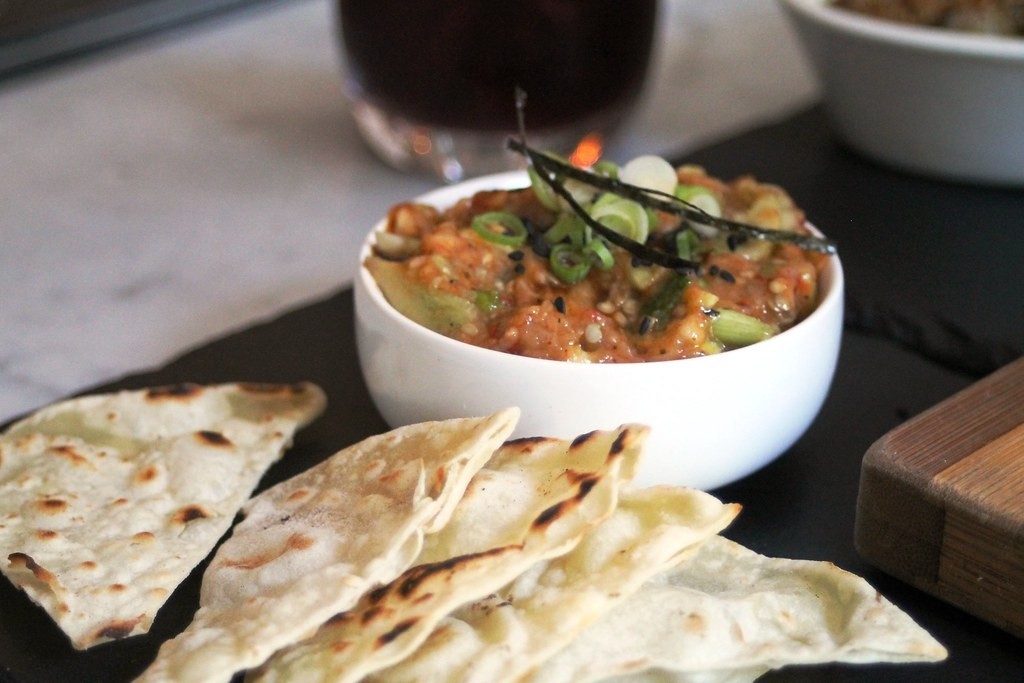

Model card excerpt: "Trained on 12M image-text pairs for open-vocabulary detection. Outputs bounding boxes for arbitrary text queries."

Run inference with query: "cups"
[324,0,675,180]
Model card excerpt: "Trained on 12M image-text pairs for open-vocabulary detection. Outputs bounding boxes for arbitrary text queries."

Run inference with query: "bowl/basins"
[353,171,844,494]
[777,0,1024,188]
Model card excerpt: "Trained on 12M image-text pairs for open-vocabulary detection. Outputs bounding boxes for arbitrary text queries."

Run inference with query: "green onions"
[471,144,777,350]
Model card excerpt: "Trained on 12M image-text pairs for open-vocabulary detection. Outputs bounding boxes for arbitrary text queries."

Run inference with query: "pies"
[0,377,948,683]
